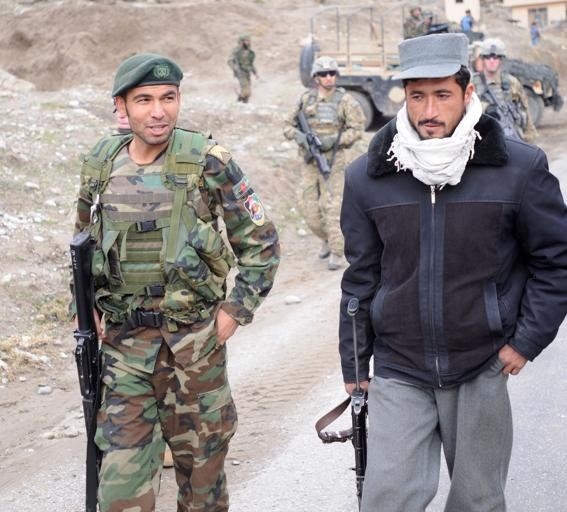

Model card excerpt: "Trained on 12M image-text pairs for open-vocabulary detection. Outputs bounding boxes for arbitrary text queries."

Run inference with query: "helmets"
[480,38,506,56]
[310,56,342,76]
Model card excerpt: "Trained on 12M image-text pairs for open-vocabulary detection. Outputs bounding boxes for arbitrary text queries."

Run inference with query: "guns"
[70,231,103,511]
[481,88,526,142]
[347,298,367,512]
[294,110,330,175]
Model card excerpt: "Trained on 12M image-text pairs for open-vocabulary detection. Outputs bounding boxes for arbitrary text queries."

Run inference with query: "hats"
[112,52,184,97]
[393,32,468,82]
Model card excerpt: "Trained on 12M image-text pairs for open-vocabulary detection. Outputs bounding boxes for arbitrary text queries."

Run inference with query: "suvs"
[298,6,563,136]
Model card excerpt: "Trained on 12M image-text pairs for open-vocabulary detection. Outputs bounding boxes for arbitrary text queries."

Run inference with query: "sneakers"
[318,241,331,257]
[328,253,343,270]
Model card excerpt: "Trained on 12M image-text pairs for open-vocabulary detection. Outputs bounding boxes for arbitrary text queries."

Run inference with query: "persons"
[400,5,422,40]
[460,9,473,33]
[470,36,539,145]
[416,10,433,35]
[336,33,567,512]
[109,98,132,134]
[282,56,366,272]
[468,41,483,75]
[530,22,540,45]
[227,35,260,104]
[71,52,281,512]
